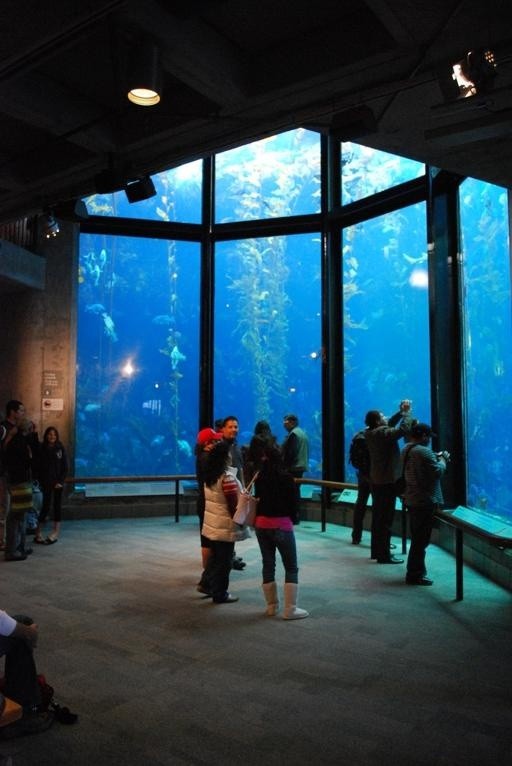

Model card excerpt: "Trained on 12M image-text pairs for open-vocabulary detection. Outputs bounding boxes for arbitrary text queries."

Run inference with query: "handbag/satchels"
[393,443,419,495]
[7,466,33,512]
[231,470,260,527]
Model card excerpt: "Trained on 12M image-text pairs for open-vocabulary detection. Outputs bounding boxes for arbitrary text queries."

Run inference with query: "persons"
[0,609,54,740]
[25,421,40,536]
[197,443,250,603]
[0,399,24,550]
[195,429,224,569]
[221,416,246,570]
[33,426,66,545]
[243,434,309,620]
[250,422,281,467]
[352,427,398,548]
[401,424,449,585]
[282,415,309,527]
[364,400,412,564]
[239,445,256,539]
[5,417,33,563]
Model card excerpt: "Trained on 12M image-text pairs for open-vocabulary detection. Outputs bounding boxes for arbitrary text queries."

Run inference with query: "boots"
[261,581,282,615]
[282,582,309,619]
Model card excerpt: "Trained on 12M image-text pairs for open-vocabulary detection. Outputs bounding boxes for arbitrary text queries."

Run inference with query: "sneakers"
[406,576,433,586]
[1,710,53,738]
[25,548,33,554]
[6,554,26,561]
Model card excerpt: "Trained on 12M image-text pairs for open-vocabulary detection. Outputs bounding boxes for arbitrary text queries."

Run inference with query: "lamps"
[127,30,165,109]
[38,213,60,239]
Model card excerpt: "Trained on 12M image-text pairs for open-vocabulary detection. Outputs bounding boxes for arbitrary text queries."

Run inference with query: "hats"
[412,422,437,437]
[197,427,223,445]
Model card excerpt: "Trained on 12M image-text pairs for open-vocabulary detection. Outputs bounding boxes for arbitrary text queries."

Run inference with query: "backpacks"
[348,432,370,472]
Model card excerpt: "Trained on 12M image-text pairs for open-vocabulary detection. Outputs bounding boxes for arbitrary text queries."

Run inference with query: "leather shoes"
[214,593,239,602]
[197,583,209,594]
[377,555,404,563]
[390,543,396,548]
[353,537,361,544]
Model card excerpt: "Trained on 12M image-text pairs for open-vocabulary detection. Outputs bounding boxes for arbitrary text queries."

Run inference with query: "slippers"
[33,536,44,543]
[44,536,58,545]
